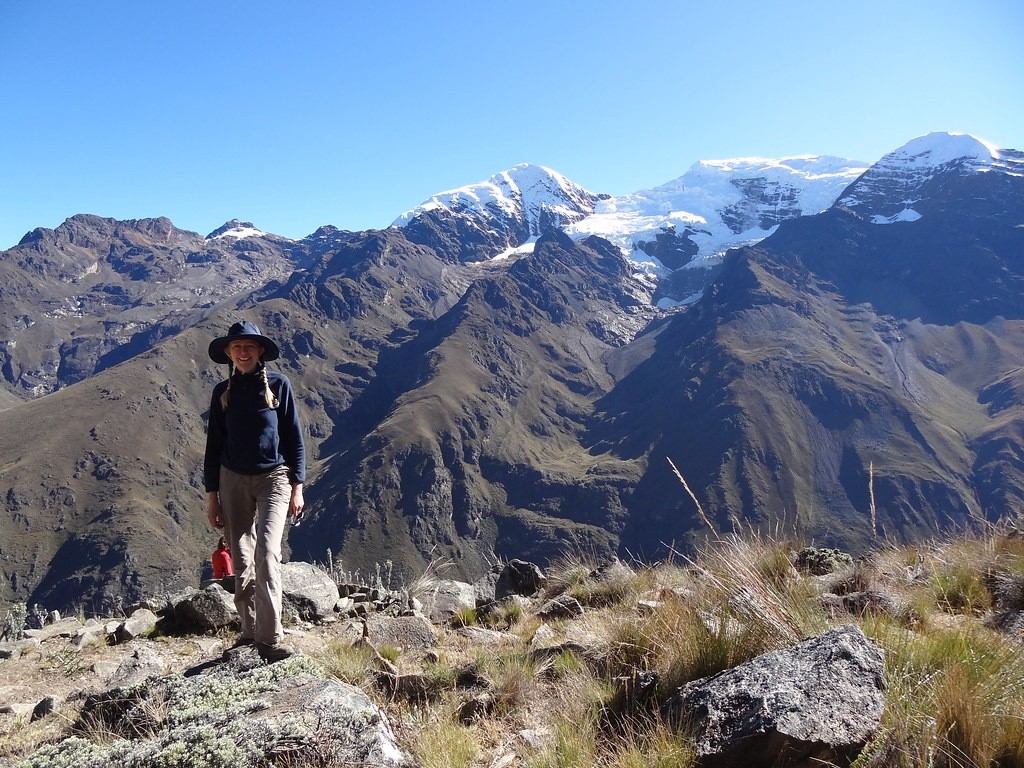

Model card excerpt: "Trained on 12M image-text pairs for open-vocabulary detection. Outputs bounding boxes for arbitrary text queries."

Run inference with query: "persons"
[205,321,304,655]
[212,536,232,579]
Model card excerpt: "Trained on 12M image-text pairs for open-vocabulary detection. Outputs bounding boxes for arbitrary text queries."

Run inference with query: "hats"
[208,321,279,364]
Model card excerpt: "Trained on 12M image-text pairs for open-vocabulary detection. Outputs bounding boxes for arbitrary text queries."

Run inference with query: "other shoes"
[259,646,290,662]
[232,639,254,649]
